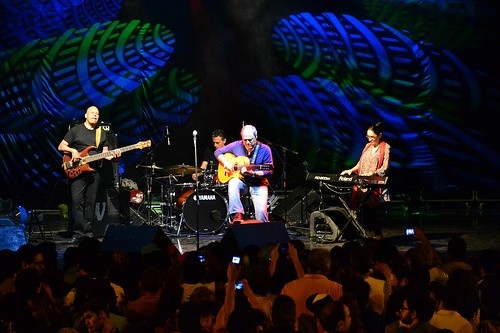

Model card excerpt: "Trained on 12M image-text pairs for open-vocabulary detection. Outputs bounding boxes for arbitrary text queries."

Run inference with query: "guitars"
[217,152,274,184]
[61,139,152,179]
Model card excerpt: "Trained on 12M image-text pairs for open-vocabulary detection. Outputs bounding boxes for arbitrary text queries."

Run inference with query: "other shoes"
[67,236,77,244]
[233,214,243,222]
[372,235,383,239]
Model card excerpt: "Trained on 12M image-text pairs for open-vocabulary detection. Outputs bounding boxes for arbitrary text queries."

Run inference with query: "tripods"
[137,170,181,233]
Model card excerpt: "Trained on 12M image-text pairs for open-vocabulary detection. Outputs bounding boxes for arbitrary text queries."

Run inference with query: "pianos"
[306,173,388,243]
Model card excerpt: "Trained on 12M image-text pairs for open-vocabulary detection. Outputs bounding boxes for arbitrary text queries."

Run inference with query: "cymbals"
[140,166,163,169]
[161,165,202,174]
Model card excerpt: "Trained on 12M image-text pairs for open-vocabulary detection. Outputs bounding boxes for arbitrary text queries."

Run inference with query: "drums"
[181,188,229,234]
[203,169,227,187]
[175,183,208,209]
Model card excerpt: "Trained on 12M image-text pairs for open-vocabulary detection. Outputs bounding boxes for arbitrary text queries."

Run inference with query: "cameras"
[235,281,243,290]
[232,256,240,264]
[403,227,415,236]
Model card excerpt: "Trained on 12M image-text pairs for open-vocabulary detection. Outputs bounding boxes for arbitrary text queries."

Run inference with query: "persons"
[192,124,275,227]
[0,227,500,333]
[339,122,391,241]
[58,105,121,244]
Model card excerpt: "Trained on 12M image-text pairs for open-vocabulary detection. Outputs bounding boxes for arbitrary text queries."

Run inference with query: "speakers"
[100,222,182,260]
[220,220,292,251]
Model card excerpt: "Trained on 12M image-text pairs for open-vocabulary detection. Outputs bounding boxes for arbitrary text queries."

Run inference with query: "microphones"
[94,123,111,127]
[192,130,198,141]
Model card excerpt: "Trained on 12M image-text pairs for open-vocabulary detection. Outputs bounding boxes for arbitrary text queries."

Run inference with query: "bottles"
[470,190,480,217]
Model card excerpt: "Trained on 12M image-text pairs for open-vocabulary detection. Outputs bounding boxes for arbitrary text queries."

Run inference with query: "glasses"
[401,305,412,312]
[365,135,380,140]
